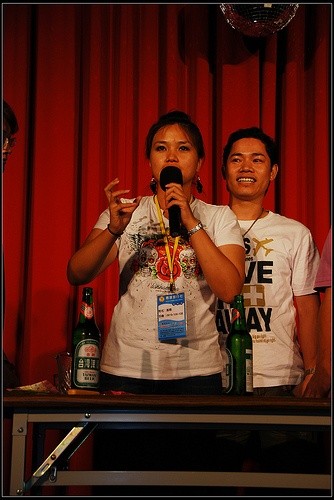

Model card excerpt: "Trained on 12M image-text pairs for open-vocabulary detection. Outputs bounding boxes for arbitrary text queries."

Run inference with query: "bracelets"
[303,367,315,375]
[108,223,124,238]
[185,218,203,236]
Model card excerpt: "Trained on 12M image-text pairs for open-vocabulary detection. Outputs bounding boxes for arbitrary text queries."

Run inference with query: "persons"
[67,111,247,472]
[312,227,333,398]
[3,101,20,174]
[214,132,321,472]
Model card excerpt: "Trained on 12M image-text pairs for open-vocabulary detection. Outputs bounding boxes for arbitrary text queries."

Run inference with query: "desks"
[3,392,331,495]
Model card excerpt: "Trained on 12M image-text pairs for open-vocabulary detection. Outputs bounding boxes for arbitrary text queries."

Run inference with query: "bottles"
[226,294,254,395]
[69,287,100,391]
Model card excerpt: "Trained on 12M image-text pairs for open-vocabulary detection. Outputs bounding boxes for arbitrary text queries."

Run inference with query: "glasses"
[3,135,17,150]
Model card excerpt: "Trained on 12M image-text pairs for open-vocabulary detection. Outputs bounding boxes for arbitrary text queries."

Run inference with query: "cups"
[56,352,72,395]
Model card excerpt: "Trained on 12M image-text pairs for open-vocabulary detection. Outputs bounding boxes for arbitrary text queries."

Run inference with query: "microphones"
[159,166,183,238]
